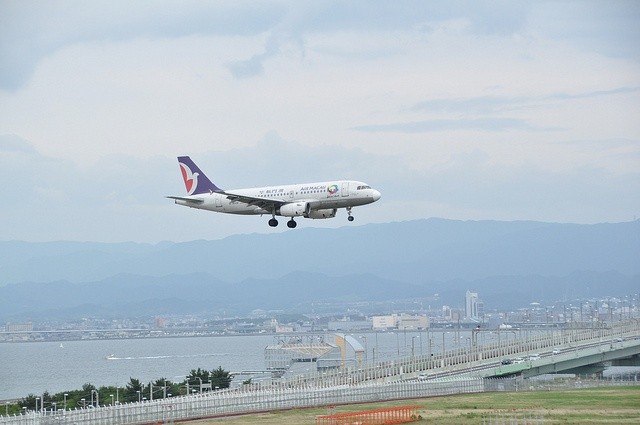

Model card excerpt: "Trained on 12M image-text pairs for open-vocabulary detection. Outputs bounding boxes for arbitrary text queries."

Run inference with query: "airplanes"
[165,156,382,228]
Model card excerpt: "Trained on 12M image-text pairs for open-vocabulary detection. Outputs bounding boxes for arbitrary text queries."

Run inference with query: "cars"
[501,358,513,365]
[553,348,561,354]
[513,358,524,364]
[530,354,540,360]
